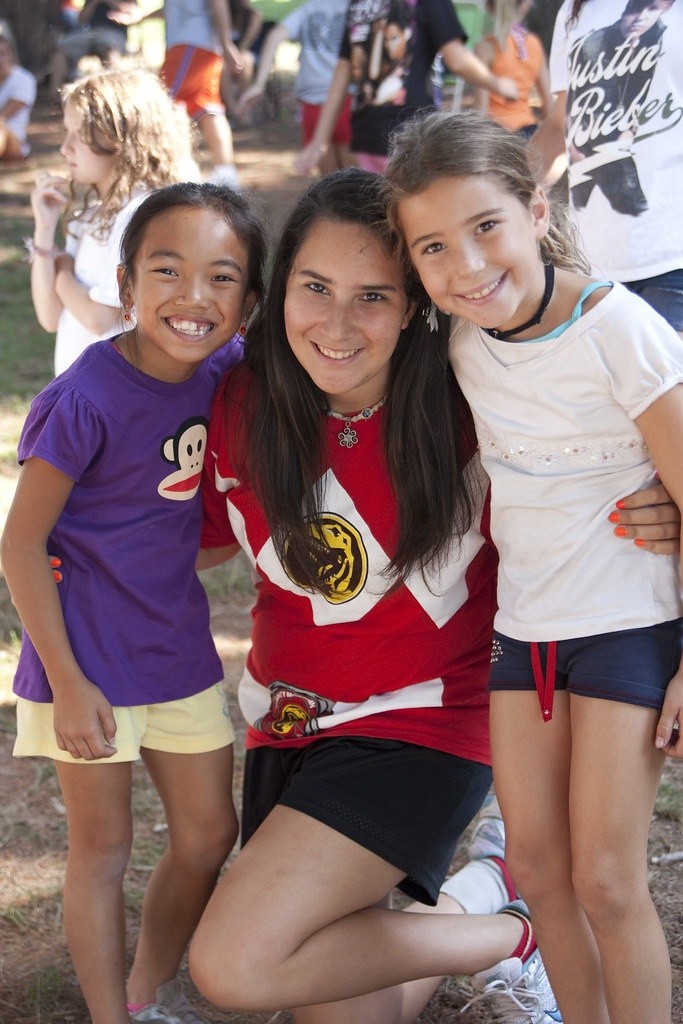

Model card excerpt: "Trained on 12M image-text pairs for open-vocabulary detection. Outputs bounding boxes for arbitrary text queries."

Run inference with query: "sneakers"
[126,975,211,1023]
[459,896,563,1024]
[462,789,508,862]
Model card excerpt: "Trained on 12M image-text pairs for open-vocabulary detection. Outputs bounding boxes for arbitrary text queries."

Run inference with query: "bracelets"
[21,235,64,265]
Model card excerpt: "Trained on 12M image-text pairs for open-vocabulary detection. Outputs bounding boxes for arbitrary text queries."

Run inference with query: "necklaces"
[487,262,555,339]
[326,394,387,448]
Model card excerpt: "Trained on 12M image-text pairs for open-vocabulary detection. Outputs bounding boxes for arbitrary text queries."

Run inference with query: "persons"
[0,181,267,1024]
[378,109,682,1022]
[233,1,360,179]
[30,68,202,377]
[160,0,242,191]
[349,0,412,110]
[524,0,683,333]
[0,0,262,139]
[289,1,518,175]
[564,0,675,217]
[46,168,682,1024]
[0,34,37,161]
[472,0,552,140]
